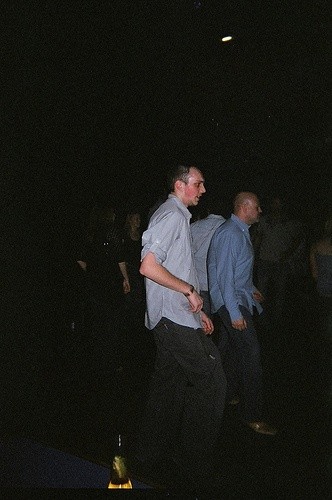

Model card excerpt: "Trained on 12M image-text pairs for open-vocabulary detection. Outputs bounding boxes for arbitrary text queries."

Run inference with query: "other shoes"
[246,420,278,435]
[225,394,239,404]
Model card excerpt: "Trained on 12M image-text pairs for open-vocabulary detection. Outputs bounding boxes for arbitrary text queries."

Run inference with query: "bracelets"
[183,285,194,296]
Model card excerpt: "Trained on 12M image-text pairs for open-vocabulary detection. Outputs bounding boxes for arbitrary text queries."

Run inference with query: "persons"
[120,208,146,347]
[131,164,226,500]
[191,192,240,405]
[206,191,277,435]
[81,202,130,388]
[251,193,310,361]
[310,214,332,297]
